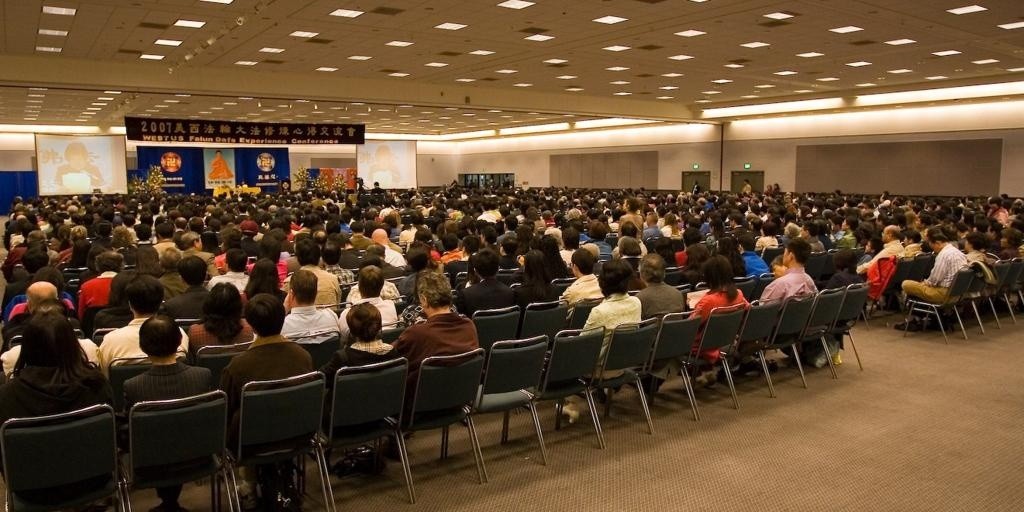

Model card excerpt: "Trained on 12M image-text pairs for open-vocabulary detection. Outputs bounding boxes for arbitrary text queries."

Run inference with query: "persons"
[741,179,752,195]
[209,150,233,179]
[366,143,402,186]
[54,140,105,192]
[691,179,700,194]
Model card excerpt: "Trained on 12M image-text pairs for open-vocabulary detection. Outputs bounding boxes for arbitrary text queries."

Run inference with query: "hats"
[241,220,258,233]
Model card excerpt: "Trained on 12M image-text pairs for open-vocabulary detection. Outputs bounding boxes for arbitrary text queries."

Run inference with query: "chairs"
[605,308,697,434]
[518,299,569,343]
[300,357,414,512]
[1,403,127,512]
[790,286,847,389]
[216,372,331,512]
[120,389,234,512]
[107,346,193,410]
[682,302,746,420]
[471,304,521,350]
[195,340,254,377]
[727,296,784,399]
[830,280,870,372]
[345,319,410,348]
[902,265,975,345]
[11,235,154,294]
[445,334,547,483]
[284,327,342,369]
[971,257,1024,334]
[386,348,483,503]
[315,249,468,320]
[760,290,819,399]
[549,233,708,301]
[567,297,606,333]
[557,317,659,449]
[504,325,603,461]
[868,251,937,327]
[4,359,101,382]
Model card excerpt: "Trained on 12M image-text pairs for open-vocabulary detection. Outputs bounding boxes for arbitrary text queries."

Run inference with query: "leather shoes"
[893,317,935,333]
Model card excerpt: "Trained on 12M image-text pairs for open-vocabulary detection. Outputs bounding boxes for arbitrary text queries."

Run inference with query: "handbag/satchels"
[807,332,844,368]
